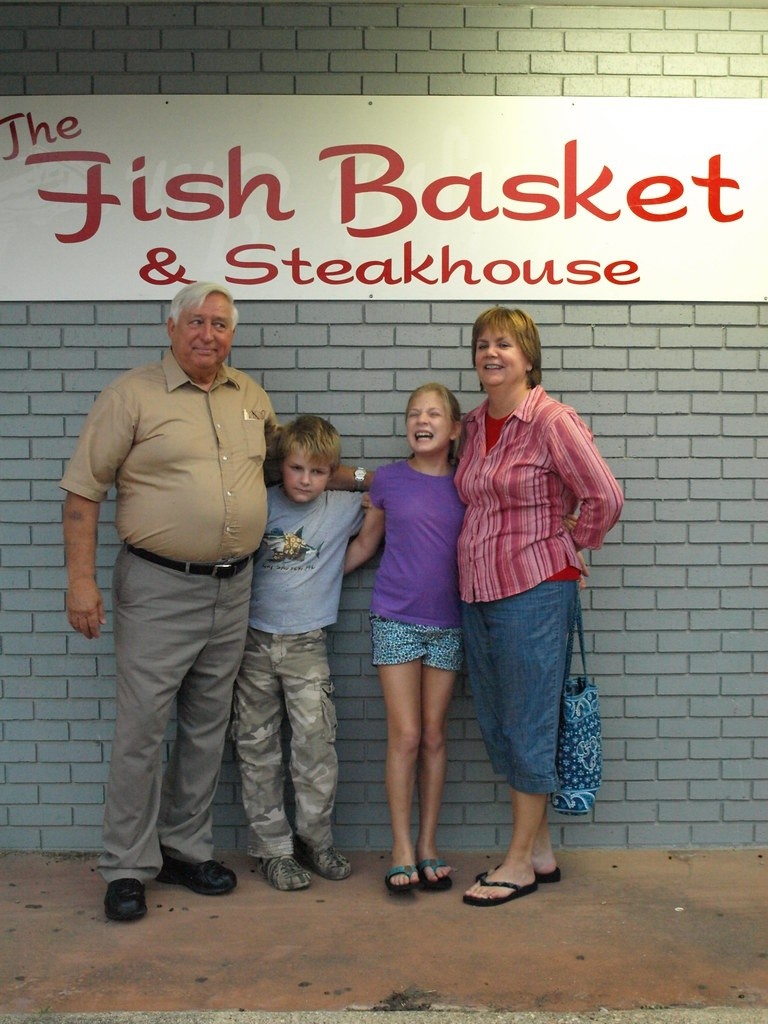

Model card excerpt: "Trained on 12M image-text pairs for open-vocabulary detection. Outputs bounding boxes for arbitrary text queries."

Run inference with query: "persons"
[57,282,377,921]
[342,383,466,894]
[453,306,625,906]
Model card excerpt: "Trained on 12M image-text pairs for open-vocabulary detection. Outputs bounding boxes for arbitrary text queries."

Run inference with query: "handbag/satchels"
[550,581,602,815]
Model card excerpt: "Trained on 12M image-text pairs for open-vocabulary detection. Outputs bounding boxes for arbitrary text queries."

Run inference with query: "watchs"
[354,466,368,490]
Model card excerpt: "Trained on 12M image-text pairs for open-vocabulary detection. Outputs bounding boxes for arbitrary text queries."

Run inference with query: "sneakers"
[256,854,311,891]
[104,877,148,920]
[299,843,351,880]
[156,853,237,894]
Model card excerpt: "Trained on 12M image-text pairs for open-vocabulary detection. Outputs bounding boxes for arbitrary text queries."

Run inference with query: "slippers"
[476,856,560,885]
[385,862,421,893]
[462,874,539,906]
[415,858,453,890]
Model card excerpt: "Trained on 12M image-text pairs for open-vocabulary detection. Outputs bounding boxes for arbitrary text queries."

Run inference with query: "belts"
[125,539,253,578]
